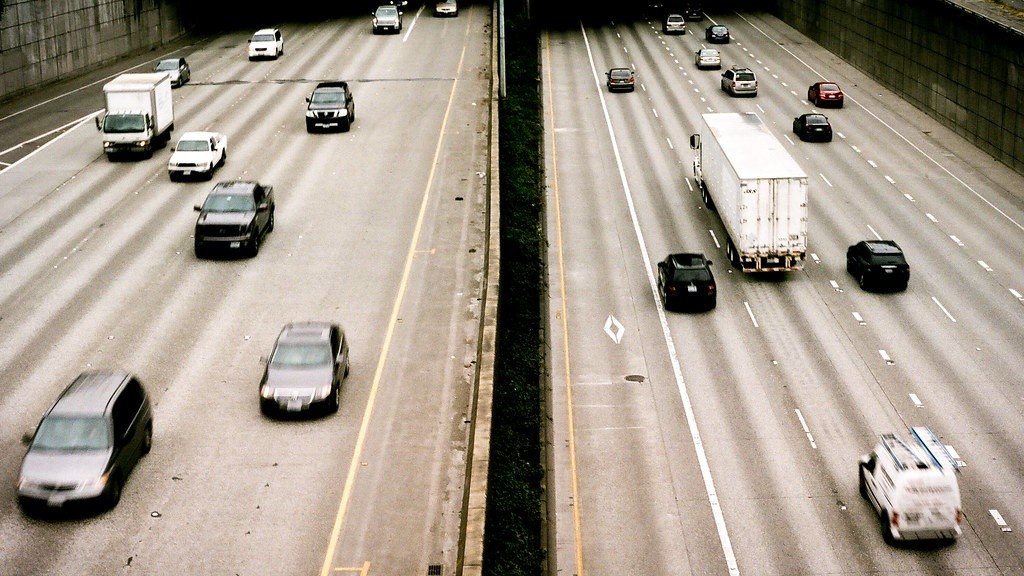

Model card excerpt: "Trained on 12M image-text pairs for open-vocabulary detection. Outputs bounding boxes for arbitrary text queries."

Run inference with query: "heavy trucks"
[689,112,810,277]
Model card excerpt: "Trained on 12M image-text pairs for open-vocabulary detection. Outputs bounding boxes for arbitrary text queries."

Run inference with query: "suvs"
[370,5,404,33]
[305,82,355,133]
[684,6,703,22]
[846,239,910,294]
[247,28,284,61]
[656,253,718,314]
[721,65,758,98]
[857,424,963,548]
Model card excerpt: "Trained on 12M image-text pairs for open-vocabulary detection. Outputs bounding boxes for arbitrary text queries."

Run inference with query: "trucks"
[95,72,174,162]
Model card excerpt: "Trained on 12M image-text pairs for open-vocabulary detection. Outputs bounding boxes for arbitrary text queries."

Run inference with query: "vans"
[16,368,154,522]
[662,14,686,35]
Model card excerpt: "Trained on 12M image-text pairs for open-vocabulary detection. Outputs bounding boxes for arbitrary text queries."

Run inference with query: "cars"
[793,114,832,144]
[695,49,721,70]
[807,82,845,108]
[258,321,350,423]
[153,57,191,89]
[435,0,457,17]
[605,67,635,93]
[705,24,730,44]
[648,1,665,11]
[389,0,411,11]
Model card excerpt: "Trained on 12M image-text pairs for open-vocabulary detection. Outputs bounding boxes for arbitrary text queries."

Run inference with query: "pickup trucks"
[168,131,228,184]
[193,180,275,260]
